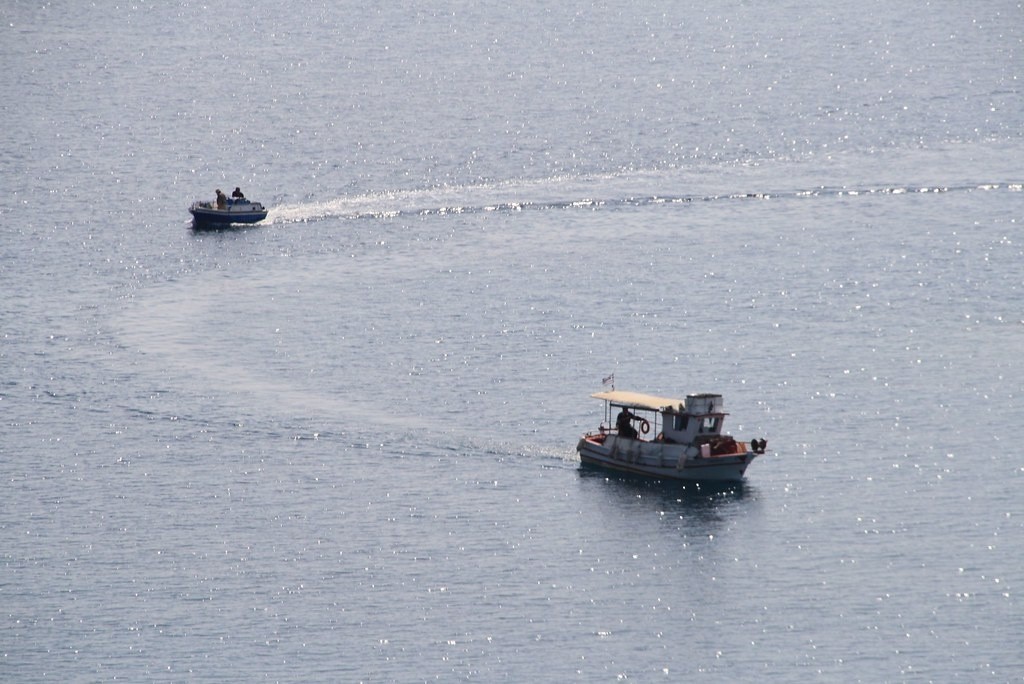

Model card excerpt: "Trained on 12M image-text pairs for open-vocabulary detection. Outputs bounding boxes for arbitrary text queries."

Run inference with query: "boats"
[575,374,767,483]
[187,197,269,224]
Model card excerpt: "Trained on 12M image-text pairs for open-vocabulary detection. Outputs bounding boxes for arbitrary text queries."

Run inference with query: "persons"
[215,188,227,210]
[616,407,643,440]
[232,187,244,197]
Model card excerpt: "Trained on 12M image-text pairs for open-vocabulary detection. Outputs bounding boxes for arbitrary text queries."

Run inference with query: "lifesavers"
[640,421,650,434]
